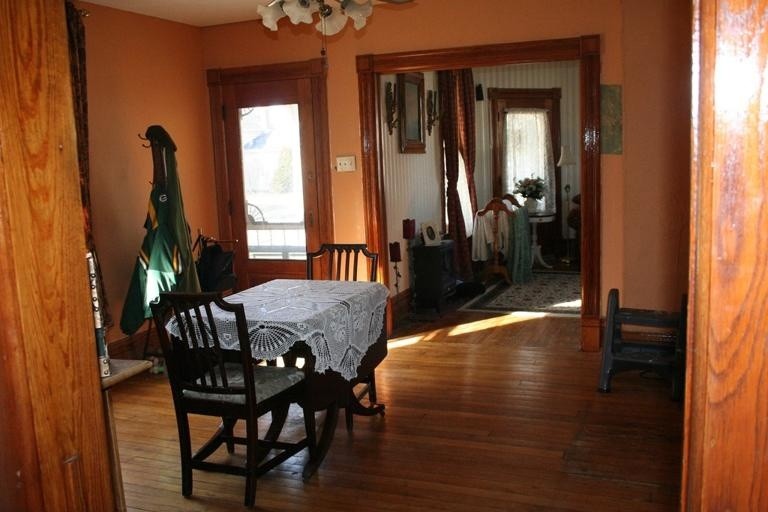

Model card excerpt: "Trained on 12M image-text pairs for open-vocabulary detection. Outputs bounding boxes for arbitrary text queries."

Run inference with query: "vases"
[524,197,538,214]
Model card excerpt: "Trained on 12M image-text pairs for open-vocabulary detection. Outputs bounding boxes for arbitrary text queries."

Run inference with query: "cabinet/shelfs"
[409,238,458,315]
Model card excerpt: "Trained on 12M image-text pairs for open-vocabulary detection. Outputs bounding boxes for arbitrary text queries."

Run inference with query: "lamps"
[254,0,373,66]
[555,144,575,264]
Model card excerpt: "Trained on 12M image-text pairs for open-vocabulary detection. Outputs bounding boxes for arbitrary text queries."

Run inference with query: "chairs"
[281,237,377,438]
[152,284,317,504]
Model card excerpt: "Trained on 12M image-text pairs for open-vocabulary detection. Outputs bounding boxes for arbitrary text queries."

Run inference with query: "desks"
[163,276,389,481]
[527,211,556,268]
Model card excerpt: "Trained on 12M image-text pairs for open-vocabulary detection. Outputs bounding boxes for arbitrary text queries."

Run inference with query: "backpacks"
[195,236,238,292]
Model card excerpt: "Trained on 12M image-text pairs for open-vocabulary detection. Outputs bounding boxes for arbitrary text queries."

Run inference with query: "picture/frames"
[396,70,427,155]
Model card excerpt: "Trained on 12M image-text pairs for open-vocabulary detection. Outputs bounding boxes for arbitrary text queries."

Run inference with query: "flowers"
[512,172,548,201]
